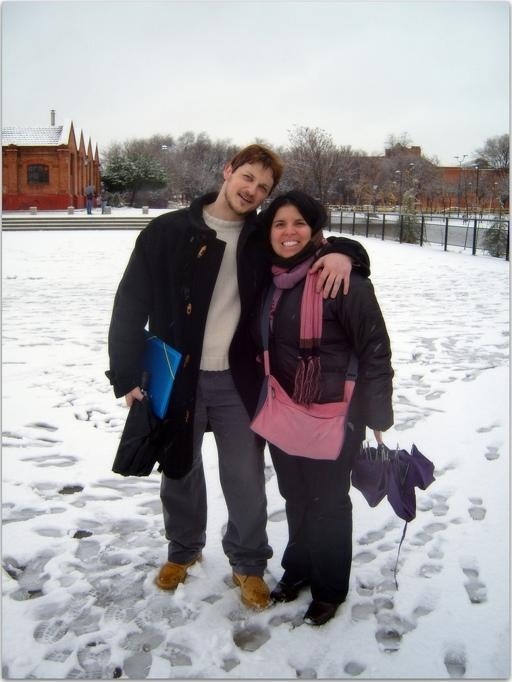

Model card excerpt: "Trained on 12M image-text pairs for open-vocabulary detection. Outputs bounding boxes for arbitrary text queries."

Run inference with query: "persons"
[86,193,93,215]
[248,188,396,627]
[105,145,371,613]
[100,196,107,214]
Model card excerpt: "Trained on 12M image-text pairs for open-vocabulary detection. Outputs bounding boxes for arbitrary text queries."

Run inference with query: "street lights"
[395,170,402,216]
[372,184,378,212]
[162,144,168,173]
[475,165,478,205]
[455,154,468,216]
[409,162,415,178]
[338,176,343,204]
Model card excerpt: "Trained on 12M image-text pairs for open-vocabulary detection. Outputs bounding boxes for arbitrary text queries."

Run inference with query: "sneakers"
[155,548,202,590]
[270,570,311,603]
[232,571,268,608]
[303,593,346,625]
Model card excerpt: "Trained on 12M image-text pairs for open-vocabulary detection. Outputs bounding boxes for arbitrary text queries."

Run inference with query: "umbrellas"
[351,440,436,522]
[112,370,165,478]
[84,185,94,196]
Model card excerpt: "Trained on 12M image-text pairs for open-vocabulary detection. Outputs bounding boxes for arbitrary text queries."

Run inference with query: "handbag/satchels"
[250,375,349,460]
